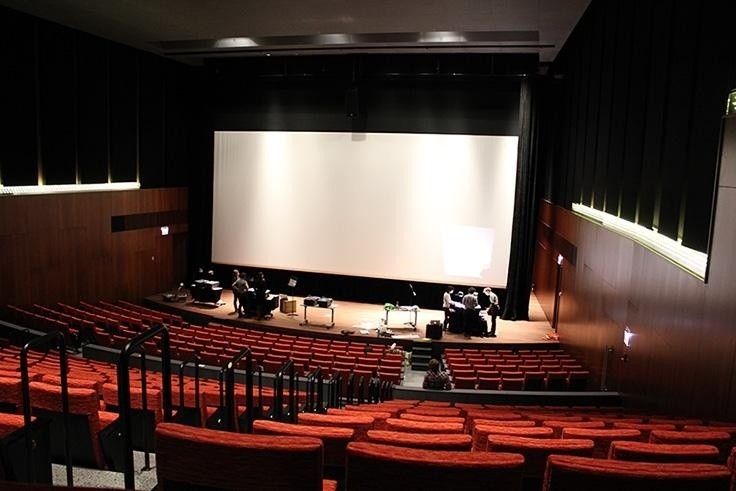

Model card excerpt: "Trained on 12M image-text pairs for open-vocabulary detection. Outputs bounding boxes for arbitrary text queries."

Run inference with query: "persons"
[232,272,249,319]
[231,269,240,313]
[423,358,452,390]
[483,287,500,336]
[462,286,478,338]
[442,285,456,332]
[254,271,267,320]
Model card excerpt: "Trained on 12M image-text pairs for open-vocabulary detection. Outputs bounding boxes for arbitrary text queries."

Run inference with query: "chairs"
[0,295,736,491]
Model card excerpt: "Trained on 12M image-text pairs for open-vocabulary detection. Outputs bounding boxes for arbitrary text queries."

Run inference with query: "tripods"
[404,284,417,325]
[287,287,299,317]
